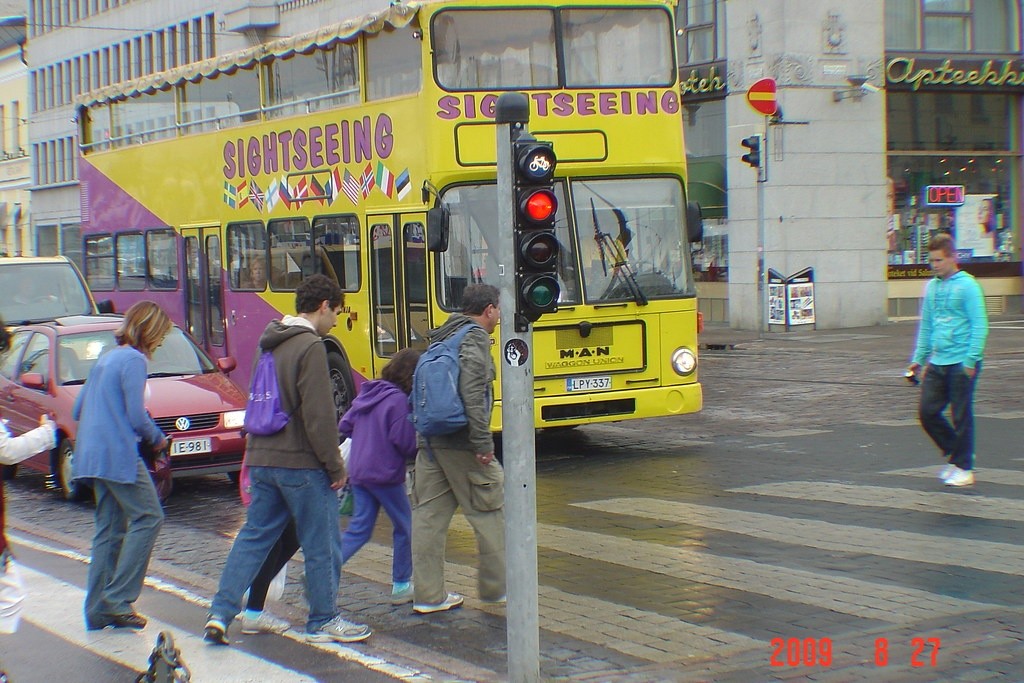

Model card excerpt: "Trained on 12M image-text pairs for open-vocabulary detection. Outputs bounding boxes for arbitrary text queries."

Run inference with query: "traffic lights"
[512,138,563,322]
[739,133,764,172]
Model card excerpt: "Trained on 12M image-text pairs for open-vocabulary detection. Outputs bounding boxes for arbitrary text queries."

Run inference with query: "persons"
[975,199,992,256]
[69,299,171,630]
[243,256,265,289]
[887,177,893,215]
[907,233,989,487]
[413,283,506,614]
[204,274,373,646]
[301,347,420,608]
[0,325,57,683]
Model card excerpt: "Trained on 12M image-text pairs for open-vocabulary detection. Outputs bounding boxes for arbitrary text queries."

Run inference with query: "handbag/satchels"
[337,434,356,516]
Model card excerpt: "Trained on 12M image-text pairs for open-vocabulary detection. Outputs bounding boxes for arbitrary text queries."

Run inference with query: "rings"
[482,456,488,460]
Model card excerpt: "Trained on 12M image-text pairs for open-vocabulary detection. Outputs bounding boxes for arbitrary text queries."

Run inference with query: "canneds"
[904,370,920,386]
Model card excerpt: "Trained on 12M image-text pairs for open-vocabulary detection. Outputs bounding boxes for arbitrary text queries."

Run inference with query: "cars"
[0,313,252,502]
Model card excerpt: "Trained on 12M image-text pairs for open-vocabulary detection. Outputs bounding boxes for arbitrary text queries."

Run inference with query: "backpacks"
[244,349,304,435]
[405,323,492,437]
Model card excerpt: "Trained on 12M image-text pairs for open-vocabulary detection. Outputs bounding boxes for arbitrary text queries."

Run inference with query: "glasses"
[161,335,166,341]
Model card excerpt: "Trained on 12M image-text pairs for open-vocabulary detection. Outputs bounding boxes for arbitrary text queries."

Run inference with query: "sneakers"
[413,593,464,614]
[203,611,230,645]
[241,610,292,634]
[305,616,371,643]
[481,594,507,607]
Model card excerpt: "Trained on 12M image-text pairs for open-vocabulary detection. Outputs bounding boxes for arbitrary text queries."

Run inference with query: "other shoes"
[100,613,147,629]
[88,618,125,631]
[940,464,957,479]
[944,466,974,486]
[390,581,415,605]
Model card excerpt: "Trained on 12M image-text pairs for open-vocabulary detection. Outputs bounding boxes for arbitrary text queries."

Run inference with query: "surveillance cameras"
[861,82,882,93]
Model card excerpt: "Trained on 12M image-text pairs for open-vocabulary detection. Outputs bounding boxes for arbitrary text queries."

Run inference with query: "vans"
[0,254,104,362]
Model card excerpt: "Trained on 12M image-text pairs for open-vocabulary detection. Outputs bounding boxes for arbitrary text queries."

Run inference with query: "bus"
[76,0,707,443]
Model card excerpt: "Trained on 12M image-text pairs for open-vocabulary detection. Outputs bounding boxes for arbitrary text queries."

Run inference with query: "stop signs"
[747,77,780,118]
